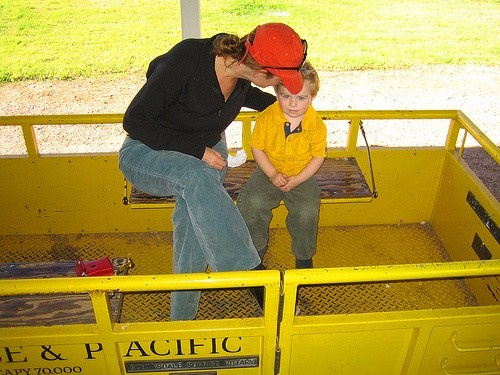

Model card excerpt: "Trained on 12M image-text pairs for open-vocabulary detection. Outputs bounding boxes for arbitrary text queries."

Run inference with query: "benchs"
[123,118,378,205]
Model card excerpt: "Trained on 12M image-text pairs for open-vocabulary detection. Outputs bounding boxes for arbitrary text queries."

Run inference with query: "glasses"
[260,38,307,72]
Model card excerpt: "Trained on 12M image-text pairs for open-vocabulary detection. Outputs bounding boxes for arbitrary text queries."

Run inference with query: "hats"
[245,23,304,95]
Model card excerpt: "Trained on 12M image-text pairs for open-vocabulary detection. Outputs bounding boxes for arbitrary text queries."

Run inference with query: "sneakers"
[246,264,300,318]
[295,258,313,269]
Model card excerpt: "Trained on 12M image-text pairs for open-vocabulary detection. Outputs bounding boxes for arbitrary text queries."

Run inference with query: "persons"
[118,22,308,321]
[237,61,328,268]
